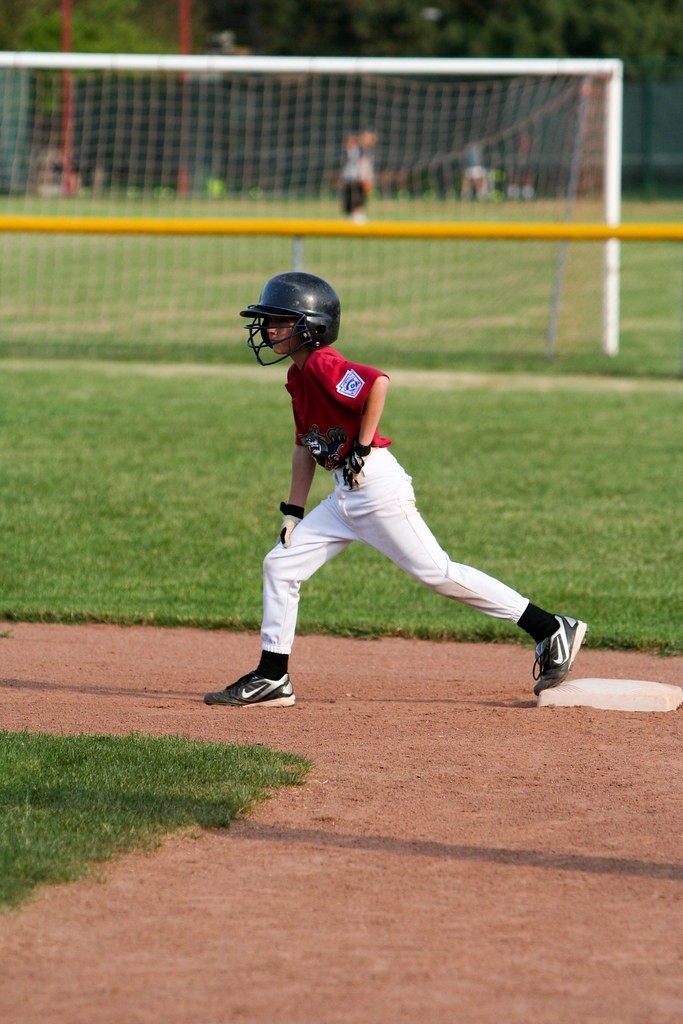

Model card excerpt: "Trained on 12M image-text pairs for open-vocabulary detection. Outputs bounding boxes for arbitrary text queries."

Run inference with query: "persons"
[339,128,376,221]
[461,138,487,200]
[202,272,587,707]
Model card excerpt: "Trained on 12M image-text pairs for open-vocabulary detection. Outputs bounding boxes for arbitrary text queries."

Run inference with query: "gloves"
[274,502,304,548]
[342,436,372,490]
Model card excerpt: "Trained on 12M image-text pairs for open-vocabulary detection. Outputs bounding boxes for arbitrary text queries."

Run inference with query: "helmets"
[240,271,340,349]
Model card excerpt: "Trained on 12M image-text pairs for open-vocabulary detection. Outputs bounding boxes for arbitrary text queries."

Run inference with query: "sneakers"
[204,671,295,709]
[533,614,590,695]
